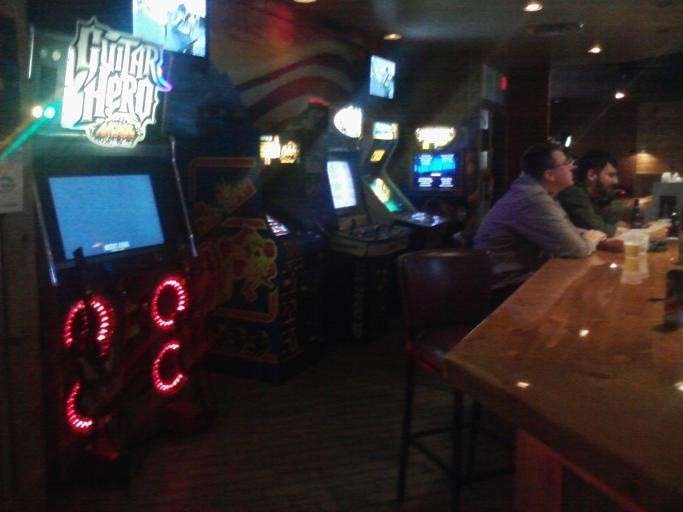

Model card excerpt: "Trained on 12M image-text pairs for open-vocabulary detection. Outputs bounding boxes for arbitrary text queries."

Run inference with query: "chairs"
[394,246,491,512]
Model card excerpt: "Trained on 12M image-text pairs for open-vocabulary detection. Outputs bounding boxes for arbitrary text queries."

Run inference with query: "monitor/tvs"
[408,149,462,196]
[368,54,399,103]
[30,147,199,288]
[104,0,210,61]
[360,168,415,214]
[322,156,366,216]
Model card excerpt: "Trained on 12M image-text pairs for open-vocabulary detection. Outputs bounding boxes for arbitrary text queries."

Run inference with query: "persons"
[557,150,672,253]
[472,140,628,298]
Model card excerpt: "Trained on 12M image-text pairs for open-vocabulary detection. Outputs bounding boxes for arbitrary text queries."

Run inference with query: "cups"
[630,228,652,279]
[620,233,644,285]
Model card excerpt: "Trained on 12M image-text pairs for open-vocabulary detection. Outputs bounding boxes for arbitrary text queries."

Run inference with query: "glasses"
[542,155,575,170]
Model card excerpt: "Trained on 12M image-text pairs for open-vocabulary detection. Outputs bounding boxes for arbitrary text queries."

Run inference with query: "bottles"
[632,200,643,229]
